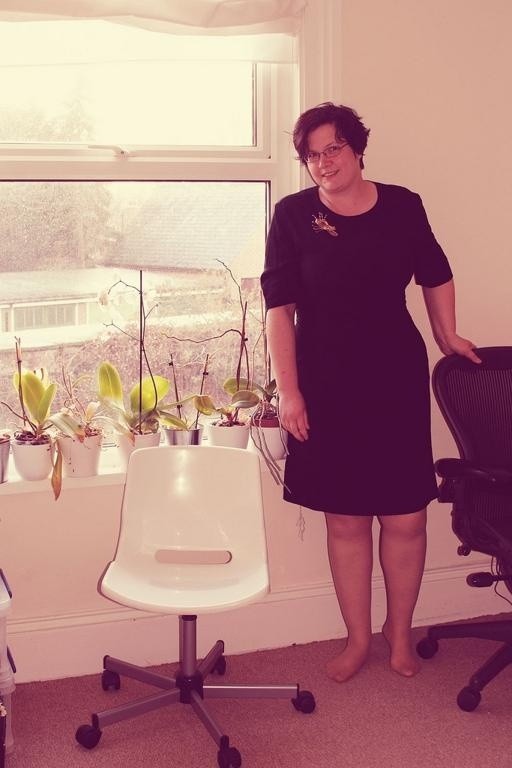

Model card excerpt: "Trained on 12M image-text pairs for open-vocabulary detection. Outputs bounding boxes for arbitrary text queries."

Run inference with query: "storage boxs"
[1,569,22,767]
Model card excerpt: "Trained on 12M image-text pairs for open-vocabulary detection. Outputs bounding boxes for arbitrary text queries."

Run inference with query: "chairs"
[414,344,511,713]
[72,444,319,768]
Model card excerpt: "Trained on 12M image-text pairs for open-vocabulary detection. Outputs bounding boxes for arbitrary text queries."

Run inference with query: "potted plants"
[0,256,287,505]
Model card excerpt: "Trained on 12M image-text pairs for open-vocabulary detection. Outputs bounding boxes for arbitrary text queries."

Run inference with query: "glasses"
[303,142,350,164]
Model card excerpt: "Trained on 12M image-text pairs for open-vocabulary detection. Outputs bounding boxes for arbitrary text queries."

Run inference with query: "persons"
[261,103,483,683]
